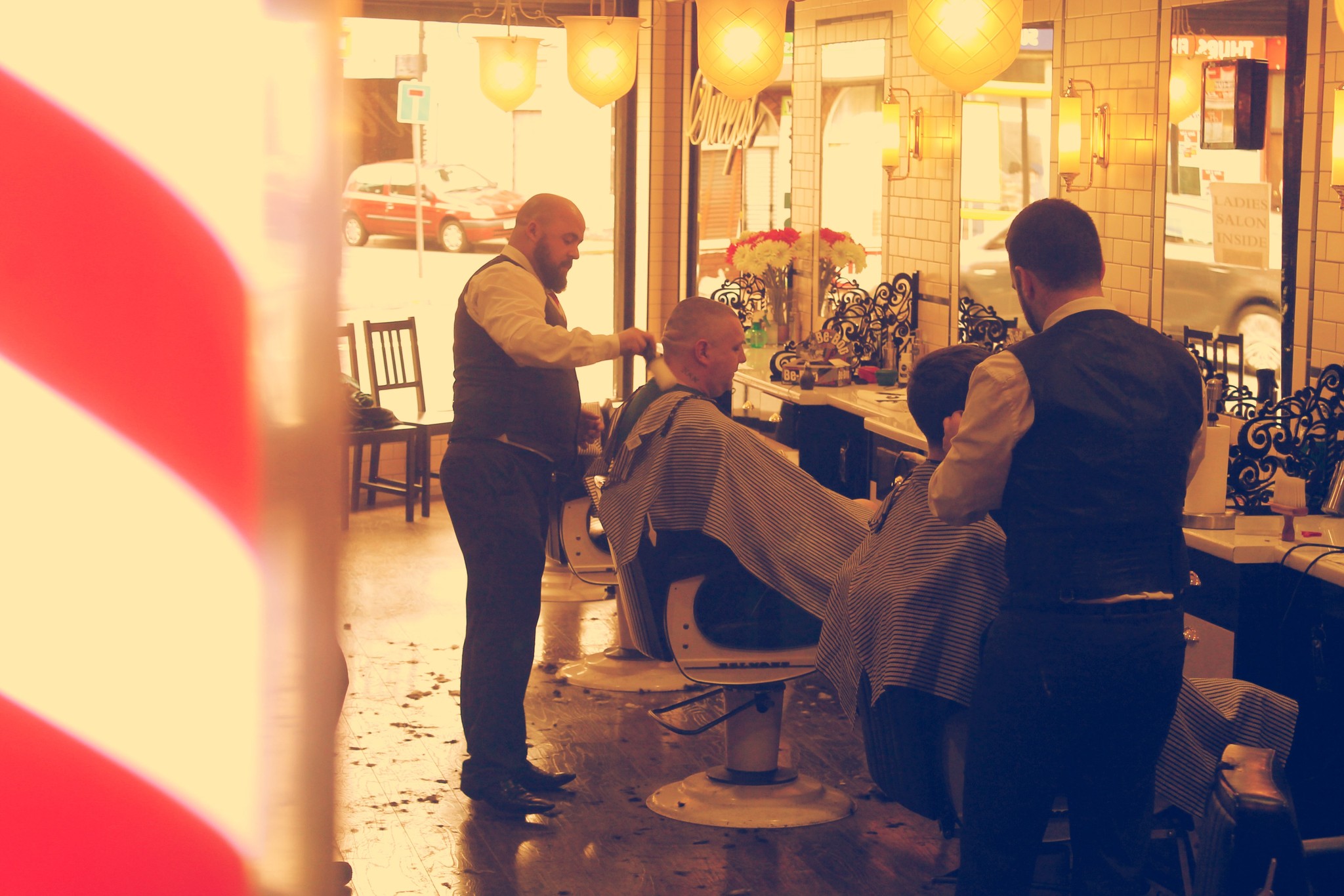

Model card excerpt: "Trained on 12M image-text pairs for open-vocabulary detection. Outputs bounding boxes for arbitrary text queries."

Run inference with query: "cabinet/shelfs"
[733,342,869,499]
[1185,546,1281,684]
[1271,560,1344,896]
[872,429,923,505]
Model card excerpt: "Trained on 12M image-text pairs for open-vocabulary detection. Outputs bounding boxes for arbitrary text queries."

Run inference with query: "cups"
[1321,452,1344,519]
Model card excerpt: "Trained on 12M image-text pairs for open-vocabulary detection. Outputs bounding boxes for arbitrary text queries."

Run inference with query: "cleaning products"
[745,309,771,348]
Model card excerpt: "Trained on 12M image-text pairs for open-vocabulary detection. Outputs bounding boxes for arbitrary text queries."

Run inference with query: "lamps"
[1058,79,1110,193]
[882,86,923,183]
[692,0,791,104]
[456,0,562,116]
[541,0,663,109]
[1330,83,1344,213]
[906,0,1025,98]
[1231,297,1283,373]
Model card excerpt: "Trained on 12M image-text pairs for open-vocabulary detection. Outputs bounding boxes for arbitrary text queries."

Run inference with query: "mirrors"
[957,20,1055,351]
[1158,0,1308,432]
[811,11,893,318]
[741,29,795,293]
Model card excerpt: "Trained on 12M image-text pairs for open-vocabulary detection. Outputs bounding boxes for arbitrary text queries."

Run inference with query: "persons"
[817,342,1301,896]
[930,195,1210,896]
[607,296,889,651]
[440,191,658,813]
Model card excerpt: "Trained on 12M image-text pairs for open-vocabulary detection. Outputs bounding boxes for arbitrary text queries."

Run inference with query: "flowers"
[818,226,867,318]
[725,228,803,326]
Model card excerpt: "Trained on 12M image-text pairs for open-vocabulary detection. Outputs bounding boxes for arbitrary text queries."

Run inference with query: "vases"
[774,323,791,344]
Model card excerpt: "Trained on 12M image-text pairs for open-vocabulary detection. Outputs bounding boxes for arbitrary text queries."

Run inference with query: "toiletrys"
[878,328,926,389]
[797,350,816,390]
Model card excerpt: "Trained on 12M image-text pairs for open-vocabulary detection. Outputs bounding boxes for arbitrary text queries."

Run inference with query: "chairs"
[1182,324,1244,417]
[544,404,709,694]
[582,471,856,828]
[842,572,1199,894]
[1198,739,1343,896]
[339,320,418,523]
[363,317,454,518]
[541,469,617,602]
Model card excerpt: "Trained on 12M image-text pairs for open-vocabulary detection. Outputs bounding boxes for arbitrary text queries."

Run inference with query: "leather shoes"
[512,759,577,791]
[461,767,556,813]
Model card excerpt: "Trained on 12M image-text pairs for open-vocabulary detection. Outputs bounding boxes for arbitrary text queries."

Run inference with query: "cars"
[339,159,532,254]
[957,220,1017,292]
[1161,189,1282,376]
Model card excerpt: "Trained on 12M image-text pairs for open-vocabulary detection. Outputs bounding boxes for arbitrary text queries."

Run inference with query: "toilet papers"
[1183,423,1231,513]
